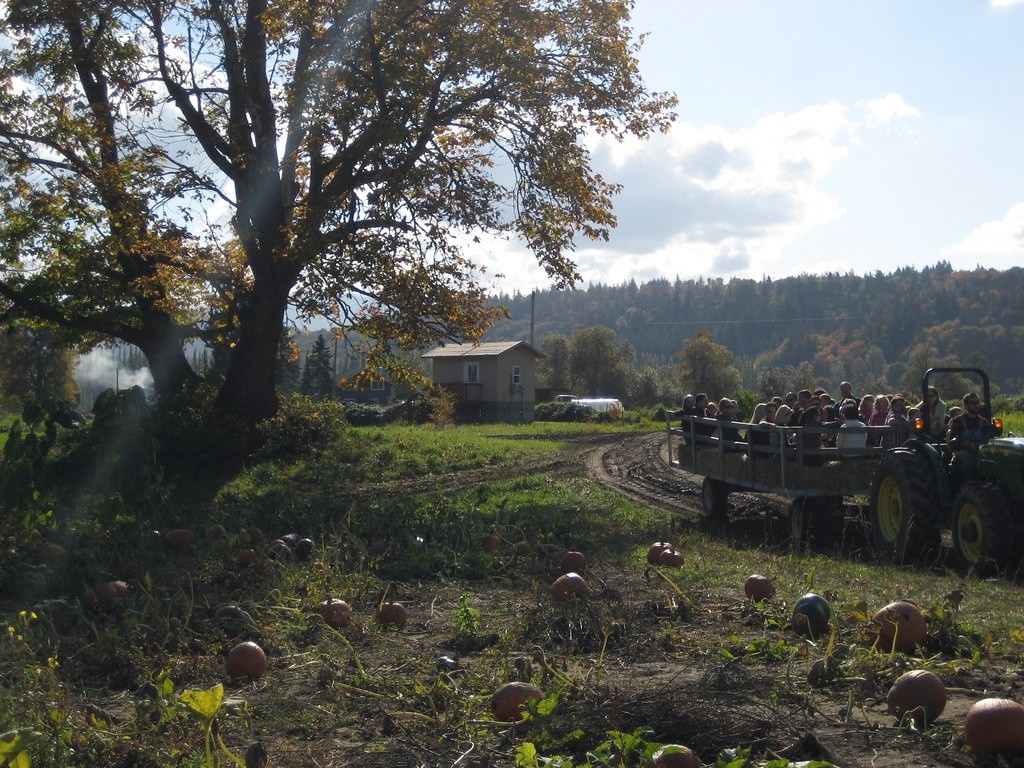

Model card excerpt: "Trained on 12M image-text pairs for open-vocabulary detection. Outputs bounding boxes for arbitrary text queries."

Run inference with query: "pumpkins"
[1,527,1024,768]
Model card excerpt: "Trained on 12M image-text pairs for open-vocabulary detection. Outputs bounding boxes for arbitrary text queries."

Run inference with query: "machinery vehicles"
[666,367,1024,577]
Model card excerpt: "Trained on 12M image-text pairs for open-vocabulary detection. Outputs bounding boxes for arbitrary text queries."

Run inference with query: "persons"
[948,392,1002,495]
[666,380,962,465]
